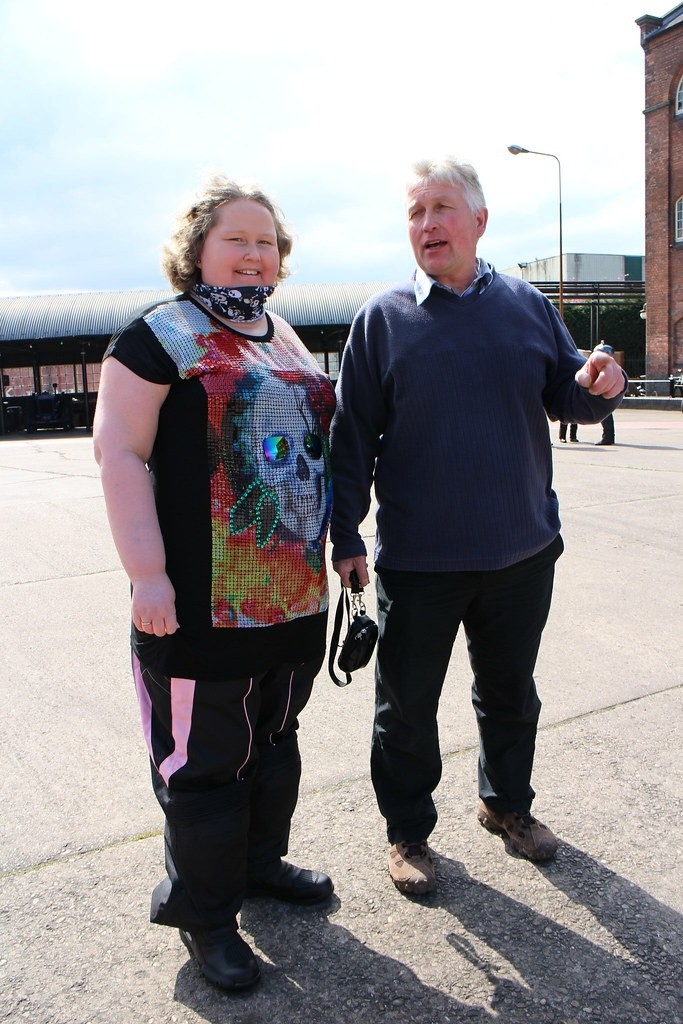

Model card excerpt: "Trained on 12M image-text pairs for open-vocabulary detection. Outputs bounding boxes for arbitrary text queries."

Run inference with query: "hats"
[593,344,614,354]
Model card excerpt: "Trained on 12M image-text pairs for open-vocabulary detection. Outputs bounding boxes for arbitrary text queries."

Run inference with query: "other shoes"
[560,438,566,443]
[595,440,614,445]
[570,438,578,442]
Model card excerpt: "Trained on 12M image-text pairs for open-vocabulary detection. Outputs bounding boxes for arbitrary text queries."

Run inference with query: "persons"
[93,186,340,997]
[328,156,627,895]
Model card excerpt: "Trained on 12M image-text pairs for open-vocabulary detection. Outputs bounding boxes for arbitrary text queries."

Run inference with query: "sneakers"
[387,840,435,894]
[476,798,558,862]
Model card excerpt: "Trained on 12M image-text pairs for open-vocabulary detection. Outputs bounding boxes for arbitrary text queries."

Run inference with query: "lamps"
[639,303,647,320]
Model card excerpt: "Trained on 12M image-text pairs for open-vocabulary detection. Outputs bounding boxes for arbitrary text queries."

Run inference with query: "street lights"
[507,144,564,322]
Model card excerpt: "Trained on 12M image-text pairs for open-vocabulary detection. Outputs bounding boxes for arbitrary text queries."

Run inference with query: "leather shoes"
[179,922,261,991]
[243,860,334,905]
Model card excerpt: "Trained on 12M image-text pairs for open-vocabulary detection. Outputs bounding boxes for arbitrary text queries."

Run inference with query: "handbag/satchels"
[328,579,378,687]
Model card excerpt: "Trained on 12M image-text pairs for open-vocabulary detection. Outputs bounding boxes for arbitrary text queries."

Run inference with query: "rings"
[141,622,152,626]
